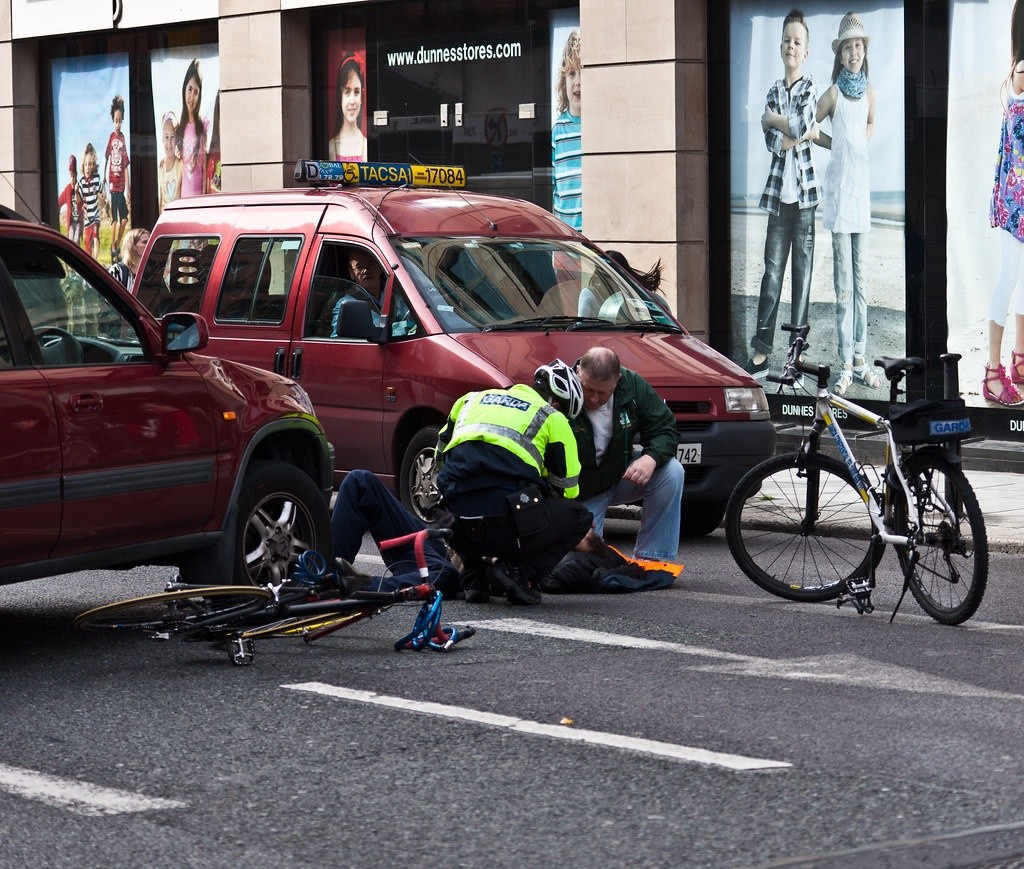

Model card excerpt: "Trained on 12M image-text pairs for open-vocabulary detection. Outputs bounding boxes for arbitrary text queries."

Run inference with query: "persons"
[551,31,582,316]
[982,0,1024,407]
[157,58,221,283]
[433,358,592,606]
[329,247,414,340]
[59,96,131,288]
[745,9,820,388]
[811,12,882,394]
[330,470,605,593]
[105,228,151,338]
[328,50,367,187]
[577,250,660,324]
[569,347,686,564]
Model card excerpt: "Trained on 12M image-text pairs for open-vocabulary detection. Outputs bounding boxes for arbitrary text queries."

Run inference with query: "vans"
[118,186,777,545]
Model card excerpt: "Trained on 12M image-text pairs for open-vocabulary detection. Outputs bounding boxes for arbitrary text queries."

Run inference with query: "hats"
[831,11,869,55]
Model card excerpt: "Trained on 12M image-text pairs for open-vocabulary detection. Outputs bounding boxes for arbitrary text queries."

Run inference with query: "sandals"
[852,365,881,390]
[835,367,853,396]
[1011,350,1024,382]
[981,364,1024,406]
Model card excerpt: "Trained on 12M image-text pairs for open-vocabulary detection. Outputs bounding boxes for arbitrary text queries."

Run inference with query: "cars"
[0,204,72,339]
[0,219,337,608]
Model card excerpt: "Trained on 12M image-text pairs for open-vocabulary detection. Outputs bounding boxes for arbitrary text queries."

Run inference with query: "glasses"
[352,263,378,270]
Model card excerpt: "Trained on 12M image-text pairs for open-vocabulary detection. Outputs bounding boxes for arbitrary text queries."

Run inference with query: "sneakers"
[464,574,490,601]
[486,560,542,605]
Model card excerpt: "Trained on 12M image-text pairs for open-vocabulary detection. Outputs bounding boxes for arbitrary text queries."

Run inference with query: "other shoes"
[745,356,770,380]
[782,373,805,390]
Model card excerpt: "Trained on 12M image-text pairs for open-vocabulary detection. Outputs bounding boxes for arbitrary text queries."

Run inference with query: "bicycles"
[722,324,990,626]
[74,528,477,666]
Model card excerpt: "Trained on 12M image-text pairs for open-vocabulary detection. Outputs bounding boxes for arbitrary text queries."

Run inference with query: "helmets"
[533,358,584,422]
